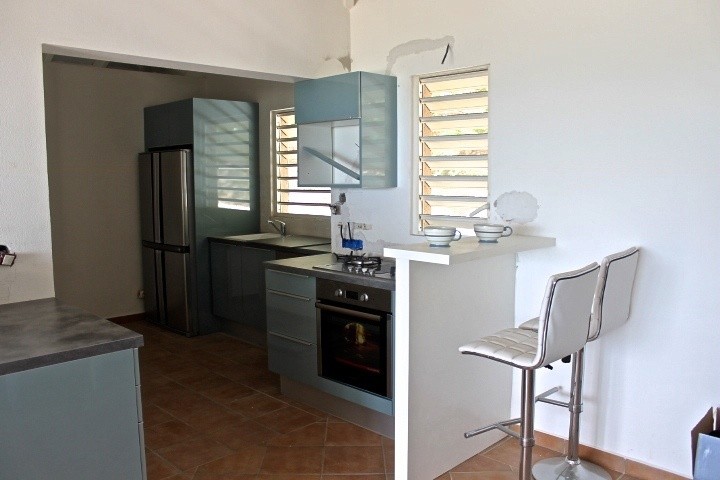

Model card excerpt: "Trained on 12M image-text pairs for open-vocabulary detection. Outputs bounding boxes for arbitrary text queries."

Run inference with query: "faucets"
[266,220,287,236]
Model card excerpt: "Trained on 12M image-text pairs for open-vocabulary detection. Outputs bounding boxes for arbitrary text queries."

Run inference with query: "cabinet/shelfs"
[292,71,397,189]
[208,240,317,386]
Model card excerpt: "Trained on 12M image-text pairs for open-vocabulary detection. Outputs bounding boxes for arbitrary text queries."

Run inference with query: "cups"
[423,225,462,246]
[474,223,513,244]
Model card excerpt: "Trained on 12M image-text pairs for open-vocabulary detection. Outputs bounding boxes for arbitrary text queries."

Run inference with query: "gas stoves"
[312,250,401,280]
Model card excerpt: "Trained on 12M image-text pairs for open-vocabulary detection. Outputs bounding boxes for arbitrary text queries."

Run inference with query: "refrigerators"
[137,147,196,337]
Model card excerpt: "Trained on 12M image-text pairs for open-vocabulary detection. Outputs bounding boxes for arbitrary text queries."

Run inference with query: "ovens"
[314,277,394,402]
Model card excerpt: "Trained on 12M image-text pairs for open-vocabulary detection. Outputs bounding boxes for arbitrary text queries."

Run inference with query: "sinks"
[218,233,291,242]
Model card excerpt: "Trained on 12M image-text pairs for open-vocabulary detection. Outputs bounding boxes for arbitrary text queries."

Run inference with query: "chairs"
[458,246,639,480]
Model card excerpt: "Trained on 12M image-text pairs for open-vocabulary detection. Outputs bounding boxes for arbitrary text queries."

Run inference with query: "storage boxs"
[690,407,720,480]
